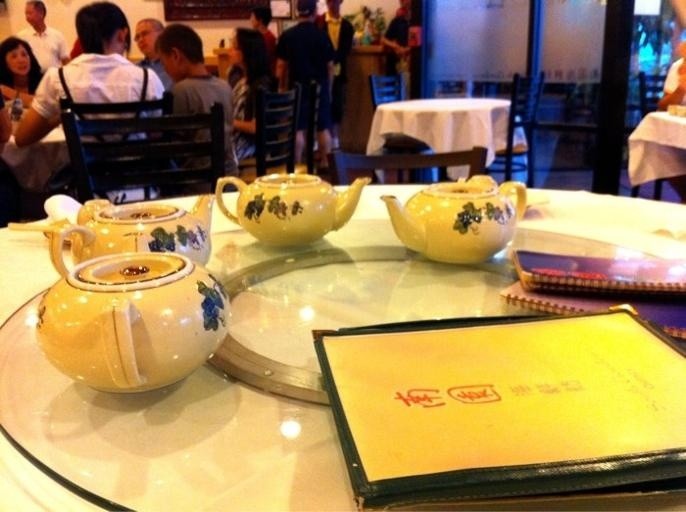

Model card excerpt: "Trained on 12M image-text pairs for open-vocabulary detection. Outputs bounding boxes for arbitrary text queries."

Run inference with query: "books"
[499,200,686,343]
[306,300,686,512]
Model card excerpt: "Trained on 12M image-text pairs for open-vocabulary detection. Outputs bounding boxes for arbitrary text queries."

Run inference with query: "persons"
[381,7,414,87]
[1,2,238,205]
[227,0,355,168]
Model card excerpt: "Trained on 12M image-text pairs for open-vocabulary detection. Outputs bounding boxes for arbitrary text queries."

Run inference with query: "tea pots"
[379,173,531,268]
[49,193,220,285]
[214,172,374,247]
[34,253,232,394]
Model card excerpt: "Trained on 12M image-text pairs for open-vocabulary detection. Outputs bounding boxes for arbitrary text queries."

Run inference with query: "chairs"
[59,73,669,198]
